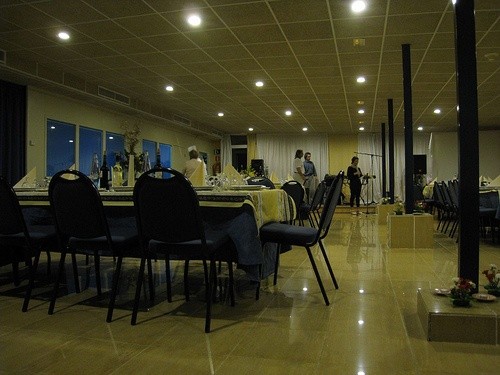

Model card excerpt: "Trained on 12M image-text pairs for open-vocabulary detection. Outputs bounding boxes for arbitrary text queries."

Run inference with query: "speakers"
[251,159,264,175]
[413,154,426,174]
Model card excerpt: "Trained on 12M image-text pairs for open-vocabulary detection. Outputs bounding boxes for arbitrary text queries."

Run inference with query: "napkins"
[188,159,205,187]
[288,173,294,181]
[271,171,278,182]
[12,167,37,188]
[61,163,76,180]
[224,163,239,179]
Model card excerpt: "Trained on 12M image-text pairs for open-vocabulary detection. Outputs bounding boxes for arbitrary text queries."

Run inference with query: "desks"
[16,189,297,286]
[387,213,434,249]
[377,205,395,224]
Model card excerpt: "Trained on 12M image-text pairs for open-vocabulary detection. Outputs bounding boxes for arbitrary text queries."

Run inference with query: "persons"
[293,150,307,203]
[347,157,364,215]
[185,145,207,187]
[303,152,317,205]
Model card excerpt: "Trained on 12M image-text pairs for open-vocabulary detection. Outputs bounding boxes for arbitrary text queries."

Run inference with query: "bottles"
[100,154,110,191]
[91,153,100,190]
[154,154,162,178]
[112,153,123,190]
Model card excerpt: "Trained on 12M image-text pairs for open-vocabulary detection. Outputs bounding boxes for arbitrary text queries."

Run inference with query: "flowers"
[381,197,391,203]
[114,118,148,173]
[394,197,403,212]
[415,200,427,210]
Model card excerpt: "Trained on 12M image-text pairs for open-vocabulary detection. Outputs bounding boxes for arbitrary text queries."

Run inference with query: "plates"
[472,294,496,301]
[112,186,134,192]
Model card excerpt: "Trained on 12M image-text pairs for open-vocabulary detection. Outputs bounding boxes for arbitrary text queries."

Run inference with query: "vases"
[395,211,402,214]
[455,299,470,306]
[482,264,500,287]
[450,278,476,300]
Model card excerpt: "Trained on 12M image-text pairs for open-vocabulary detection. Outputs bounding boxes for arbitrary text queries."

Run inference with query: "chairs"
[0,167,345,334]
[425,179,500,244]
